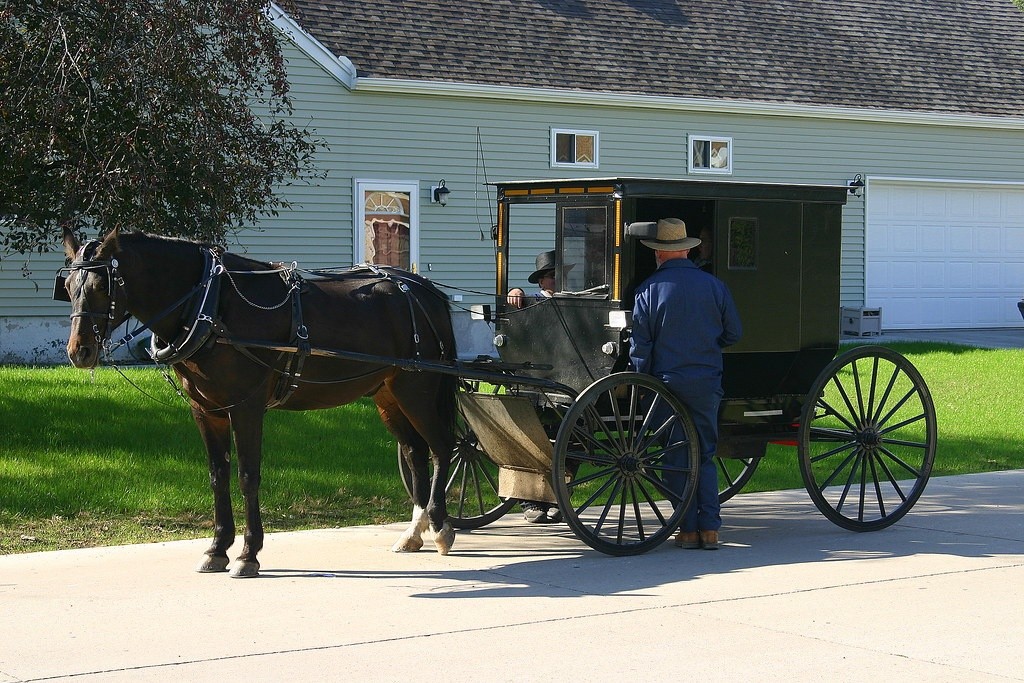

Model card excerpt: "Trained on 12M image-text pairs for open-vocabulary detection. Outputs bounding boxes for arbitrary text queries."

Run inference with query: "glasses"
[540,272,555,279]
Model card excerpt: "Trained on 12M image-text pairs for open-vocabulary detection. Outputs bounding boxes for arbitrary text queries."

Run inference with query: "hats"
[528,250,576,283]
[640,218,702,251]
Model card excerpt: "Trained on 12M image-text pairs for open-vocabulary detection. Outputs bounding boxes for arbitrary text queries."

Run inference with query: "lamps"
[850,174,865,198]
[434,179,450,207]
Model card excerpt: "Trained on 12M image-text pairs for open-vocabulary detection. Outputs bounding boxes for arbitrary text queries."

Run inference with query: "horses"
[55,221,461,580]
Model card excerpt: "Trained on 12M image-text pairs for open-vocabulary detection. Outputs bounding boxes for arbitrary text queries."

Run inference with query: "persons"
[691,223,713,276]
[628,218,734,550]
[506,251,586,523]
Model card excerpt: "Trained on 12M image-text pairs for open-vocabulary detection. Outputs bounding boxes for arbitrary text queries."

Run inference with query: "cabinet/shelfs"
[841,305,882,337]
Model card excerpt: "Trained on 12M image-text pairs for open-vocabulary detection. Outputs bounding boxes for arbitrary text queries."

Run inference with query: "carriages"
[61,176,939,580]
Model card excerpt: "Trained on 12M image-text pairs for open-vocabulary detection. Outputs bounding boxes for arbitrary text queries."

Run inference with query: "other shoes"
[675,531,700,549]
[524,506,547,523]
[547,506,562,522]
[700,530,718,550]
